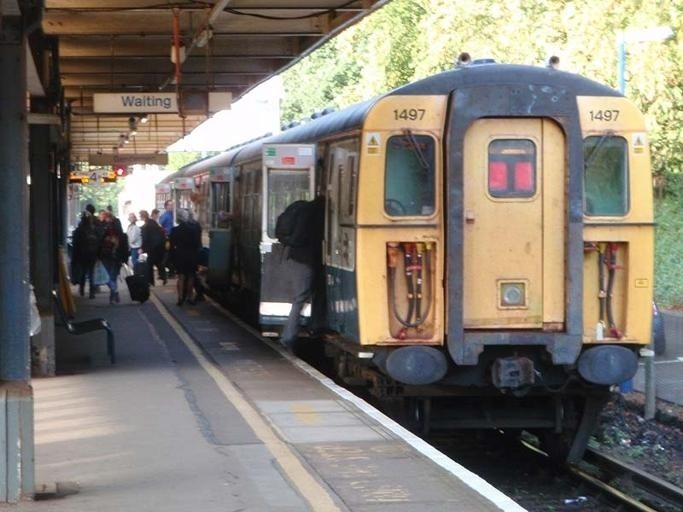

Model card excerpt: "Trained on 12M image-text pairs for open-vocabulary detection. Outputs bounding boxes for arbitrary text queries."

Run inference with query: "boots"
[177,278,205,306]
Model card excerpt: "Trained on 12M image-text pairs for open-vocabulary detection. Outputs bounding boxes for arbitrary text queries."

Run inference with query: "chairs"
[48,288,113,364]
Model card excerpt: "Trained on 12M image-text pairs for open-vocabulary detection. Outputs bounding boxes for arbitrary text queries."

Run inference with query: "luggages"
[121,260,150,303]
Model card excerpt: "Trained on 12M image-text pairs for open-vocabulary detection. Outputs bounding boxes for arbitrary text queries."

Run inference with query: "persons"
[183,206,205,303]
[274,196,325,356]
[73,196,174,304]
[168,208,200,305]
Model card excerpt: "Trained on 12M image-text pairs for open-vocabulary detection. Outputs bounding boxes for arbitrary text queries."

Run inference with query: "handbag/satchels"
[275,200,322,247]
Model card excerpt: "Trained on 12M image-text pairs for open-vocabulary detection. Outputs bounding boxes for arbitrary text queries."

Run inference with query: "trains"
[155,52,656,483]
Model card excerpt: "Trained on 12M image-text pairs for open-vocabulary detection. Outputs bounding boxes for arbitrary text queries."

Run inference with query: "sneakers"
[306,327,325,337]
[276,338,299,356]
